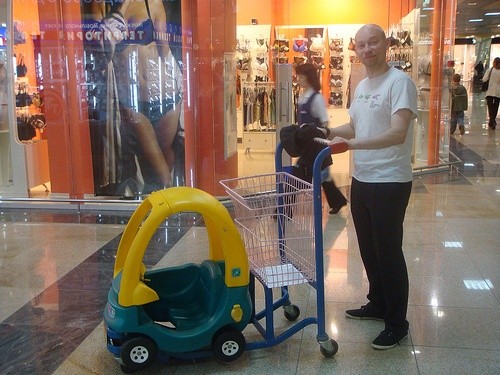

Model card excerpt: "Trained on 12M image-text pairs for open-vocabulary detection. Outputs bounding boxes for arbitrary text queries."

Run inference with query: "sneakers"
[345,301,381,321]
[371,321,409,350]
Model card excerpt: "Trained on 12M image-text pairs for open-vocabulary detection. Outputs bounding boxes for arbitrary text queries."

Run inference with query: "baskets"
[218,171,316,288]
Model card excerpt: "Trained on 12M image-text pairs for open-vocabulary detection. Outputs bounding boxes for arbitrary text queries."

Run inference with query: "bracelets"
[319,125,331,139]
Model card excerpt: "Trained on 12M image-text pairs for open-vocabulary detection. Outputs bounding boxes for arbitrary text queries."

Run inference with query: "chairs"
[170,260,222,329]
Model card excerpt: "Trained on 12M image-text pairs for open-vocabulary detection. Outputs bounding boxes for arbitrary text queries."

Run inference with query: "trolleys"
[104,122,349,375]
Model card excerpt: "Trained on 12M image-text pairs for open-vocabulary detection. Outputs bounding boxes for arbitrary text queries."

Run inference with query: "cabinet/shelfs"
[0,21,50,194]
[236,8,419,155]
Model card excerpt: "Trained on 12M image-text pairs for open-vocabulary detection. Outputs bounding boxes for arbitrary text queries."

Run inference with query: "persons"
[481,56,500,130]
[87,1,184,196]
[270,62,348,220]
[448,73,469,135]
[296,24,419,350]
[476,60,484,78]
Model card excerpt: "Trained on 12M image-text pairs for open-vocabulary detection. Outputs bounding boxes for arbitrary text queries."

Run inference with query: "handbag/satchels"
[481,67,493,92]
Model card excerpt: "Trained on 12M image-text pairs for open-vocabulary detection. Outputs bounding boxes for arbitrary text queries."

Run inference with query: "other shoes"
[329,200,347,214]
[450,125,465,135]
[488,121,497,130]
